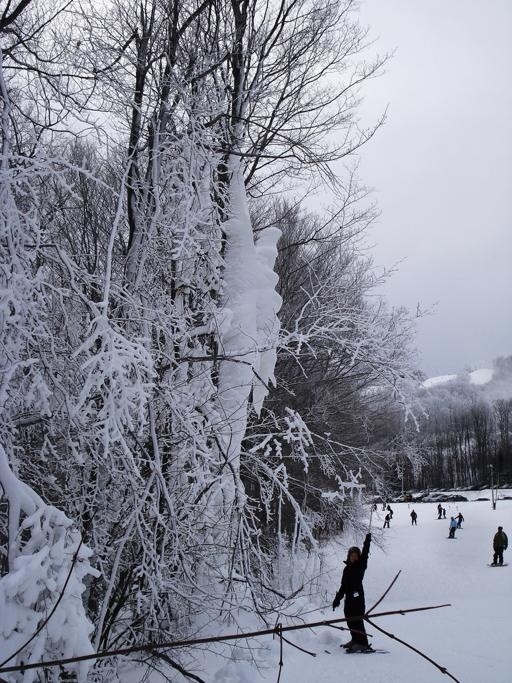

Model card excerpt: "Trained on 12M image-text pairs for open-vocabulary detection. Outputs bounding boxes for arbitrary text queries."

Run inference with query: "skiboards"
[339,644,391,653]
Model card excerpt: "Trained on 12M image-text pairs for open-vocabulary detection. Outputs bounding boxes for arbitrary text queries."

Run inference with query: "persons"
[448,516,457,538]
[442,508,446,519]
[410,509,417,525]
[491,527,508,566]
[438,504,443,519]
[333,534,371,653]
[455,512,464,529]
[372,502,393,528]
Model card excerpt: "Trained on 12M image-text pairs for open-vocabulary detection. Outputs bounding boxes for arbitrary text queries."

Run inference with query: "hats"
[346,545,361,561]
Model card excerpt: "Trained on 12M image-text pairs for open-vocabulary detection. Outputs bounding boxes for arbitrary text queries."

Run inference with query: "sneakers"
[344,638,373,651]
